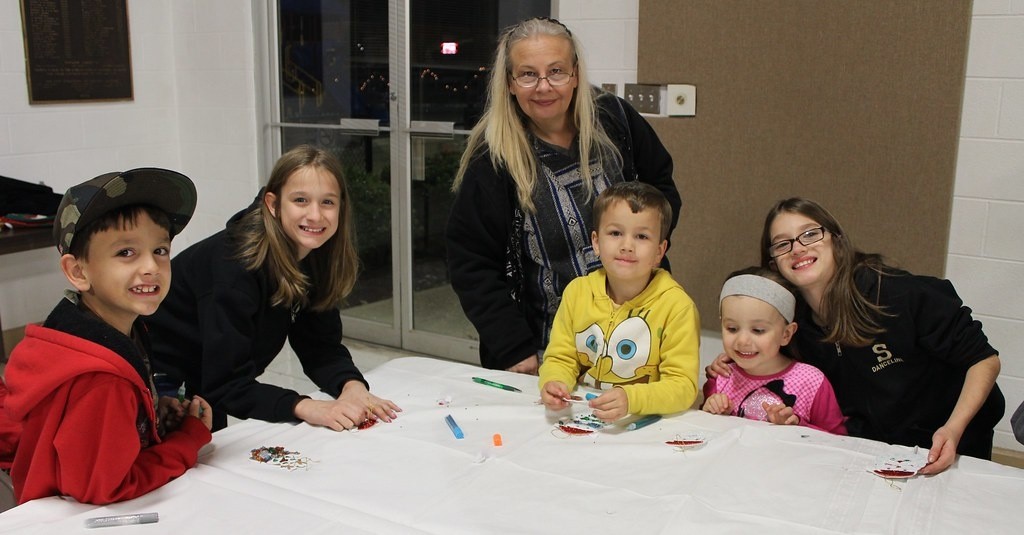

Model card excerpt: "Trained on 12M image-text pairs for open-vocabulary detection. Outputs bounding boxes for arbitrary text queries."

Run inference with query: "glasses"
[507,61,576,88]
[767,225,838,258]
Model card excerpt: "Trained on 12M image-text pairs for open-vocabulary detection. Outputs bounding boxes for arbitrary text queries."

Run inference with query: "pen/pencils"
[470,375,523,394]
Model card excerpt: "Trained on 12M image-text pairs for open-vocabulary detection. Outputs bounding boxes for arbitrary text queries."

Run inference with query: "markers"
[175,380,188,404]
[624,416,662,431]
[85,512,160,529]
[444,415,464,439]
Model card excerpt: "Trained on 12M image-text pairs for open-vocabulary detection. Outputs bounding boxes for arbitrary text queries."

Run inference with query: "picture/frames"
[19,0,135,105]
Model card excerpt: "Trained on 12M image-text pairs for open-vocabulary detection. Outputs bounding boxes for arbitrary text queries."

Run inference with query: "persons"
[0,166,213,506]
[699,196,1024,478]
[537,179,699,424]
[444,16,684,372]
[131,142,403,434]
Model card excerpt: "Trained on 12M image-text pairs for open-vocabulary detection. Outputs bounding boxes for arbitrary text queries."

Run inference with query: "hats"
[52,167,197,258]
